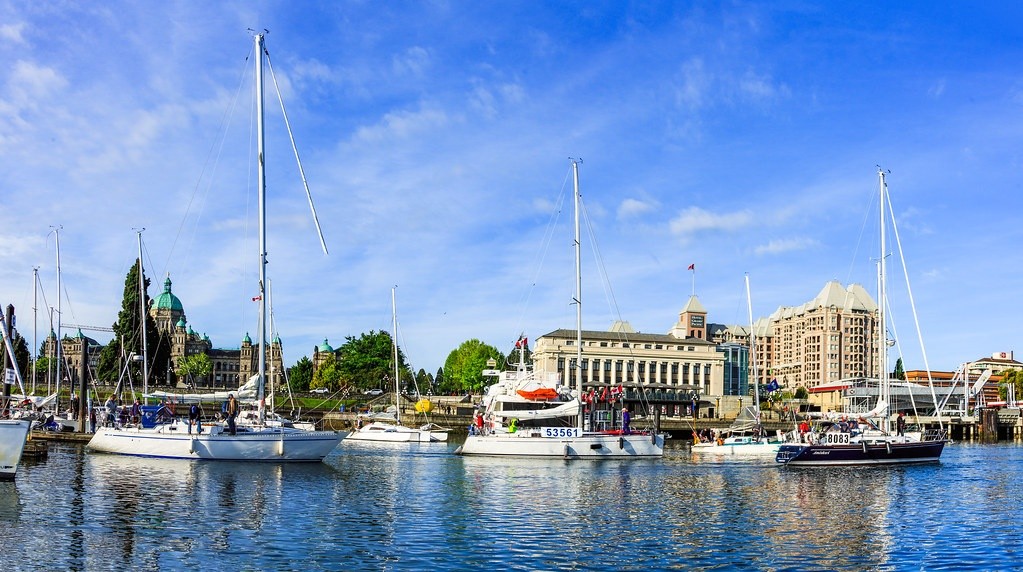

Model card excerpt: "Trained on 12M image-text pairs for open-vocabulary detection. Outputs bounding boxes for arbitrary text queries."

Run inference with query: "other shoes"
[228,432,235,435]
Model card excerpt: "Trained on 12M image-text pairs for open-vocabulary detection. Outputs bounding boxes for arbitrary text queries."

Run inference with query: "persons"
[119,406,130,423]
[897,413,904,436]
[215,394,237,436]
[611,407,617,430]
[358,416,362,429]
[477,412,484,433]
[105,394,117,427]
[90,409,96,434]
[131,397,142,423]
[188,405,201,434]
[800,420,809,434]
[622,408,631,433]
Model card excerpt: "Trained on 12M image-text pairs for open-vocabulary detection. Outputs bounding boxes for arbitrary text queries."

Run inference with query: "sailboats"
[0,24,367,481]
[774,163,955,469]
[688,270,801,459]
[346,284,455,444]
[451,154,670,460]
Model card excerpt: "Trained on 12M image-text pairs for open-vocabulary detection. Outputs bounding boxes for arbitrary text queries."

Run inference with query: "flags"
[582,384,622,404]
[765,379,778,392]
[688,264,694,270]
[251,296,261,301]
[515,338,527,347]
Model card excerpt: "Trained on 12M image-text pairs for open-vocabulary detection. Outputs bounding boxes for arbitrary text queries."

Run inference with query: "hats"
[192,406,196,413]
[228,394,233,398]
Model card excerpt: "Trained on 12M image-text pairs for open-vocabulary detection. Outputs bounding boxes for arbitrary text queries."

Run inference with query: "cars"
[364,388,384,396]
[308,386,330,395]
